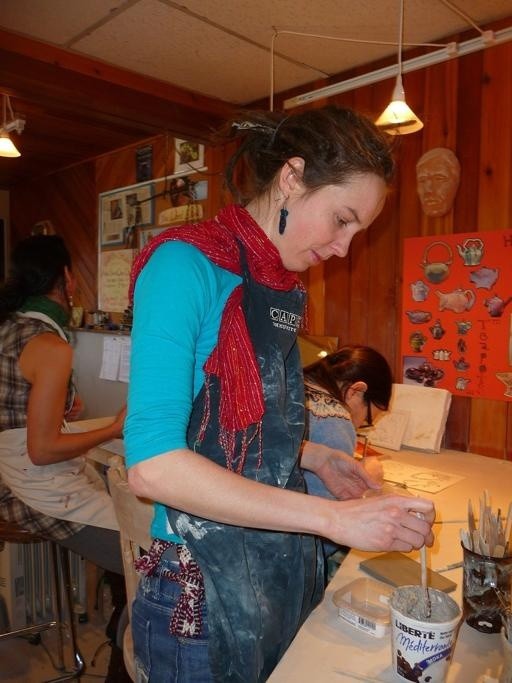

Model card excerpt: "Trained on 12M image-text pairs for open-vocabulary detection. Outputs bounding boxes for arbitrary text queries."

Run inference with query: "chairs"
[103,453,165,683]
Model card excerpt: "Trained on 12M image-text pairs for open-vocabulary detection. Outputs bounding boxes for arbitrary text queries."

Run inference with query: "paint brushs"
[417,499,432,622]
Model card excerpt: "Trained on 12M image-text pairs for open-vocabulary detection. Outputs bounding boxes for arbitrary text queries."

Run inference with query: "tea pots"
[456,238,484,266]
[404,309,431,324]
[419,242,453,284]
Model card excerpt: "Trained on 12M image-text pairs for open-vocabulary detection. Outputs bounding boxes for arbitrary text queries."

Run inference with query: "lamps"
[0,93,21,158]
[366,0,425,136]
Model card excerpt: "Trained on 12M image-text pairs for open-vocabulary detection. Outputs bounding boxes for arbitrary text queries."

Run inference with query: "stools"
[1,526,85,683]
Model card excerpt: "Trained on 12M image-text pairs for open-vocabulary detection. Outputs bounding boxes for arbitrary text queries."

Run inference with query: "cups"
[460,540,512,633]
[388,584,462,683]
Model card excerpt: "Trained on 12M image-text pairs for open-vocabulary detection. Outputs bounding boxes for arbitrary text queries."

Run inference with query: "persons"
[0,231,133,683]
[122,102,439,683]
[414,144,461,215]
[304,342,394,558]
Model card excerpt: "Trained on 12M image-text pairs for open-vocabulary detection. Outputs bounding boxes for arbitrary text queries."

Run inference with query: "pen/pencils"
[363,436,368,457]
[459,490,511,558]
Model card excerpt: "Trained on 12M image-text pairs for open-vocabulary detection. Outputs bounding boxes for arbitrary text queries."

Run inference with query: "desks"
[62,414,512,682]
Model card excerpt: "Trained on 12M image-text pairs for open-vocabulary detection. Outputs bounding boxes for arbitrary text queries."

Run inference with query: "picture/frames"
[174,136,205,174]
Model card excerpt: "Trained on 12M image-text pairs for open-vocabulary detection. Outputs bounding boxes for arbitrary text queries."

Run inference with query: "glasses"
[357,393,375,432]
